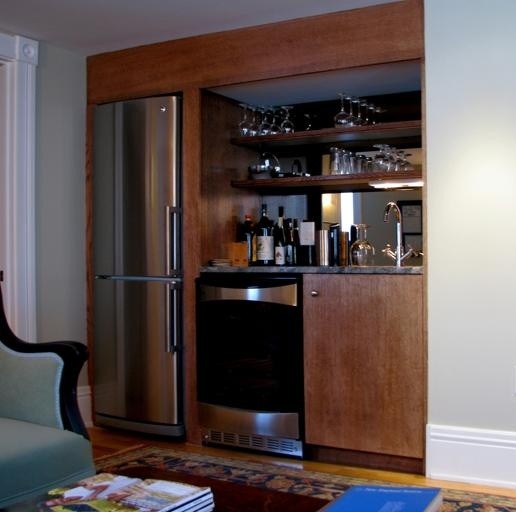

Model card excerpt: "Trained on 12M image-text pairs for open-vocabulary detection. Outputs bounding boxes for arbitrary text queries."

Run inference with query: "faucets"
[381,201,418,266]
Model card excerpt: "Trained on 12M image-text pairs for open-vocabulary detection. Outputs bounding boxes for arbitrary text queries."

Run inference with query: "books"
[316,483,445,511]
[2,471,216,512]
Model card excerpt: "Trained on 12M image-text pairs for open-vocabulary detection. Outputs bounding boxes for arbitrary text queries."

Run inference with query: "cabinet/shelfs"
[86,0,425,478]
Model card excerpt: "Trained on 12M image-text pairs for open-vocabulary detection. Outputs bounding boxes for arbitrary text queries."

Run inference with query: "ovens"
[193,272,302,455]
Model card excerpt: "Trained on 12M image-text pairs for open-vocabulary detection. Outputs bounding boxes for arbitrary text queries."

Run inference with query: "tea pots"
[248,150,283,181]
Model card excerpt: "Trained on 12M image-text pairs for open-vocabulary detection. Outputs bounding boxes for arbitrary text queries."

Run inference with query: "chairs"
[0,290,95,512]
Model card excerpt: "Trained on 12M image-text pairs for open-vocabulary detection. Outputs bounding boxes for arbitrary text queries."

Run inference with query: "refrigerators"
[90,90,181,437]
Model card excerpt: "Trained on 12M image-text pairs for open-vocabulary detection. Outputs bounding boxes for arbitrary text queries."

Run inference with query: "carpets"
[0,440,516,512]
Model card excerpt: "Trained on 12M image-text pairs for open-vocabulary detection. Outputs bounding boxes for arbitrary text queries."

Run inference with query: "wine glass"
[237,89,385,137]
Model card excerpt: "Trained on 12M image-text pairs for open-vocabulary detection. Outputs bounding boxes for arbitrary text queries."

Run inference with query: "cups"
[328,145,417,175]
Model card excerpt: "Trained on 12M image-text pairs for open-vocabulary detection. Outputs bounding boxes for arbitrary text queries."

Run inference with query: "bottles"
[234,204,360,267]
[351,225,377,267]
